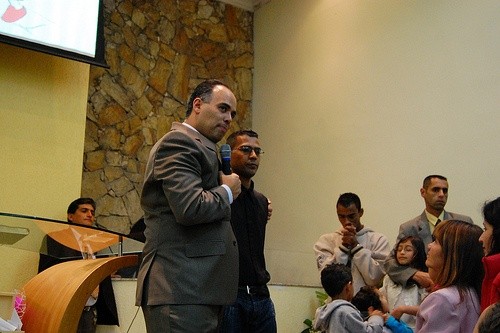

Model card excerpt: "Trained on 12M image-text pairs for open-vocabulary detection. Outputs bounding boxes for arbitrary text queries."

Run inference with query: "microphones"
[221,144,231,175]
[95,223,113,254]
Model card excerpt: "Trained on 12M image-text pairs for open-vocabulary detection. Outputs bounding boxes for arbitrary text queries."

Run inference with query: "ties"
[81,238,99,300]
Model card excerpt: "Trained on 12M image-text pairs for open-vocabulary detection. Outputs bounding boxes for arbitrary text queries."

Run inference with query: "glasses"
[234,147,265,156]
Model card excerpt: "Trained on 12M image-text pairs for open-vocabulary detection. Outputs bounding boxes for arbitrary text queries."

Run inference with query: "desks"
[0,213,147,333]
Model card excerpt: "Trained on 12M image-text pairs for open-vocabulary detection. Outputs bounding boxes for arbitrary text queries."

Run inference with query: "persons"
[384,175,474,288]
[227,130,278,333]
[311,263,384,333]
[390,220,485,333]
[477,197,499,315]
[36,197,120,333]
[132,80,273,333]
[312,193,391,298]
[351,285,414,333]
[378,235,428,333]
